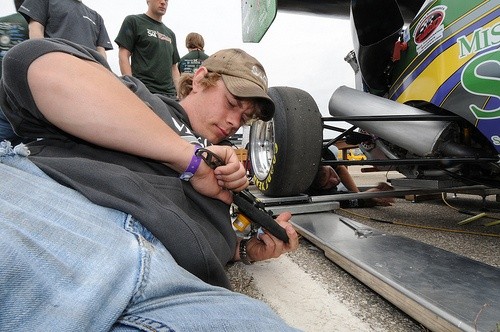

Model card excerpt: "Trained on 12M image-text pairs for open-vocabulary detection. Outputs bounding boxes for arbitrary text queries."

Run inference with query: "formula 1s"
[240,1,500,199]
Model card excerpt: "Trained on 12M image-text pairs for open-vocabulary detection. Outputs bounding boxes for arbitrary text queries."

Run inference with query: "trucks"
[337,148,366,162]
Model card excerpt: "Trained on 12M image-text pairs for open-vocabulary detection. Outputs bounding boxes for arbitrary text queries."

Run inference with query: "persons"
[17,1,113,64]
[177,32,209,76]
[0,40,274,332]
[114,0,180,102]
[309,142,394,209]
[0,0,30,77]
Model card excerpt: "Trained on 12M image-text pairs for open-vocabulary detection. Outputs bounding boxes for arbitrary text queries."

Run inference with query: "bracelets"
[181,143,201,181]
[239,236,256,266]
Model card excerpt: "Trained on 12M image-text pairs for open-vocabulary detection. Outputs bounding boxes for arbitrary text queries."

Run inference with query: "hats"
[199,48,275,122]
